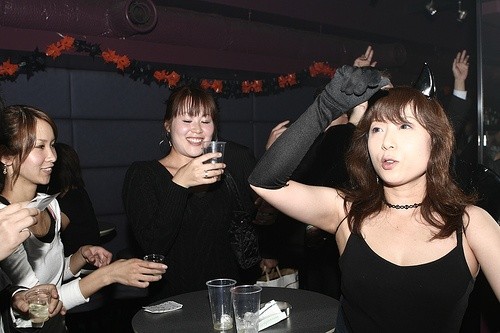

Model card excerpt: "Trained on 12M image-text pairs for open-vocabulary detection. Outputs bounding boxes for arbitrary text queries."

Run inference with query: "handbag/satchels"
[254,265,300,289]
[222,171,262,269]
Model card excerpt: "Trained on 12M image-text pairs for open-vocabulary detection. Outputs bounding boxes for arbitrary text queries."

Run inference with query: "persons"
[248,65,500,333]
[0,104,168,333]
[123,84,279,308]
[264,45,469,301]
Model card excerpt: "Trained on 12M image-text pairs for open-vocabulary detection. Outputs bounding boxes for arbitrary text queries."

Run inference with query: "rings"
[204,170,207,178]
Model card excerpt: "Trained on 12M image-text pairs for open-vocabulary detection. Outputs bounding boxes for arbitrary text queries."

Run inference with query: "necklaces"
[383,199,422,210]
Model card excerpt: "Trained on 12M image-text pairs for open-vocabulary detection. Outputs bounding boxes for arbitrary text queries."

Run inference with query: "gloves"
[246,64,390,189]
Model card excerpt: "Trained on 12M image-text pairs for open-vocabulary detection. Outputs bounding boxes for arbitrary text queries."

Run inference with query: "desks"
[131,286,339,333]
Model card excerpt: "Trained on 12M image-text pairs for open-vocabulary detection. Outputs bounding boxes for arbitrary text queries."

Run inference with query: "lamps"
[455,0,472,24]
[424,0,437,16]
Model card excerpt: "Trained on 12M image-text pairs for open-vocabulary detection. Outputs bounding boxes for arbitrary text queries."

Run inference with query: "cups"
[201,141,228,182]
[144,254,164,283]
[25,290,51,323]
[229,285,262,333]
[206,278,237,331]
